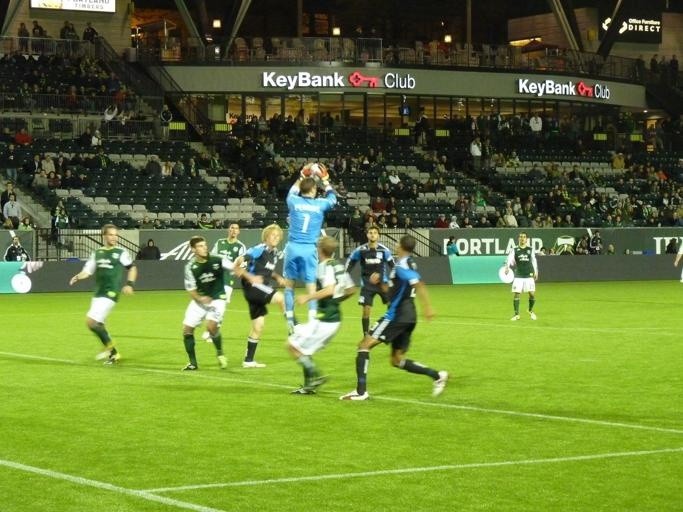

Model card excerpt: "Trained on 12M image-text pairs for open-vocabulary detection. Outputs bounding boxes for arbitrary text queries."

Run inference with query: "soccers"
[302,163,321,184]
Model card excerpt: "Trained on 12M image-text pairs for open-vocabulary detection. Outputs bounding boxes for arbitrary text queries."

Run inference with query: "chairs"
[0,34,683,234]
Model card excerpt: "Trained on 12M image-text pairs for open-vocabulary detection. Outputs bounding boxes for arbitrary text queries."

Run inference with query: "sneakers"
[182,364,197,370]
[339,390,368,400]
[242,361,265,368]
[290,376,325,394]
[433,371,448,395]
[511,315,520,320]
[96,350,119,366]
[527,310,536,320]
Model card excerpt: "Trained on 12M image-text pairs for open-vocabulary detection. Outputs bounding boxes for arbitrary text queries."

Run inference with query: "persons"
[338,234,449,401]
[345,225,397,340]
[71,225,140,365]
[282,163,338,334]
[284,235,355,395]
[0,19,683,228]
[140,239,161,260]
[2,236,32,262]
[232,223,286,368]
[178,235,230,370]
[539,231,683,284]
[201,222,247,341]
[504,231,539,321]
[446,235,460,256]
